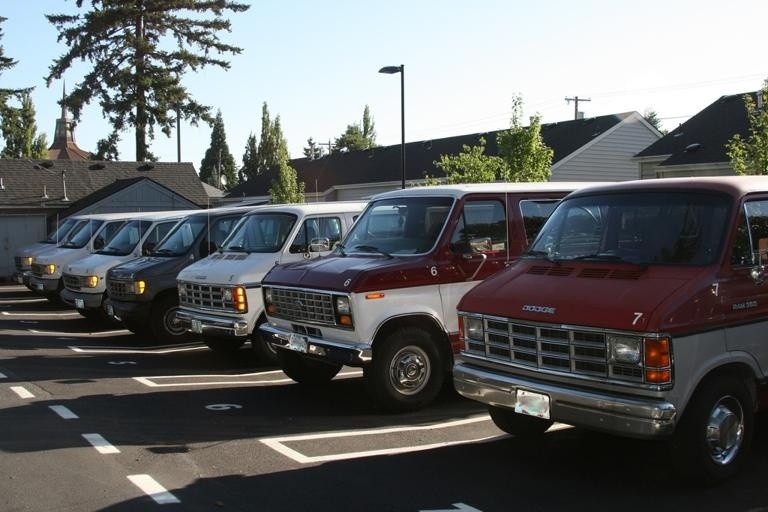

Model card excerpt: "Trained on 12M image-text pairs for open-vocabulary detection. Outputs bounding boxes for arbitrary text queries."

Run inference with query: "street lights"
[379,65,406,188]
[169,104,180,163]
